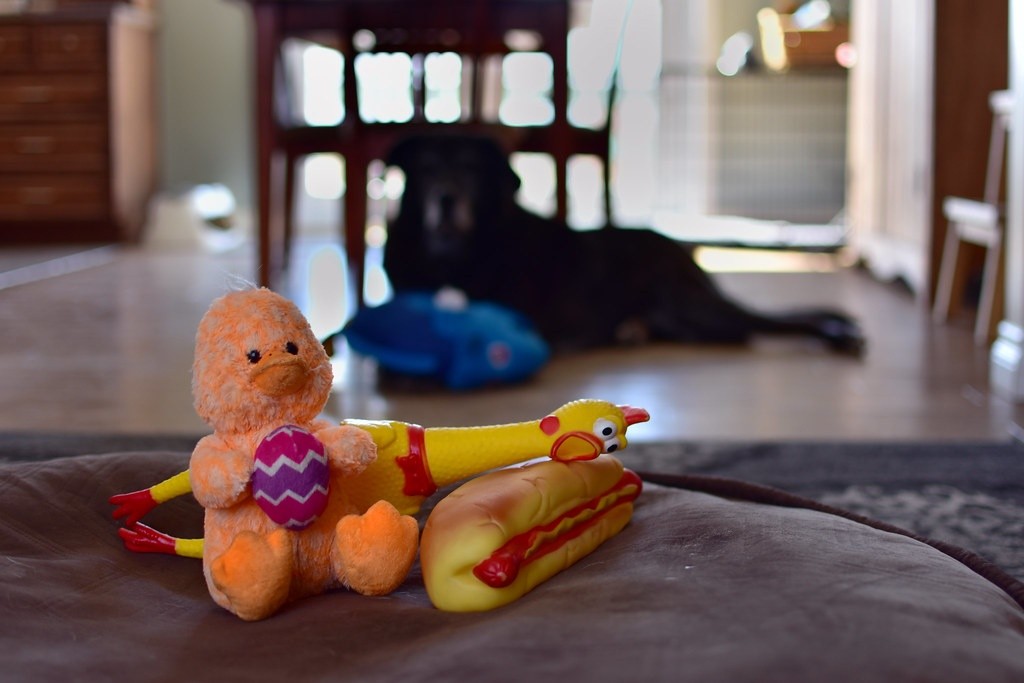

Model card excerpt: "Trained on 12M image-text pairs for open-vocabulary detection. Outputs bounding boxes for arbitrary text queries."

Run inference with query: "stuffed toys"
[189,283,419,620]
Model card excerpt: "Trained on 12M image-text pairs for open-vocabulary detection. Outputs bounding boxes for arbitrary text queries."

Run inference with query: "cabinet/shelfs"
[2,0,158,250]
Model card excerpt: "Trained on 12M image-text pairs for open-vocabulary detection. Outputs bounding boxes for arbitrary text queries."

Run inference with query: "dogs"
[375,132,864,392]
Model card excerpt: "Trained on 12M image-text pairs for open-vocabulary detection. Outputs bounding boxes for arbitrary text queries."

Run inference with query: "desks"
[249,0,626,290]
